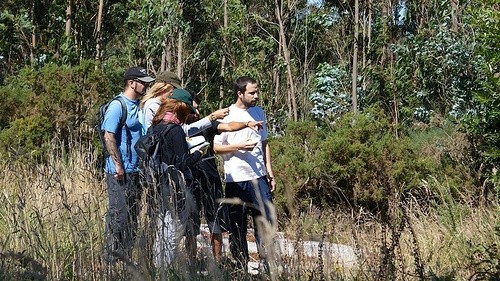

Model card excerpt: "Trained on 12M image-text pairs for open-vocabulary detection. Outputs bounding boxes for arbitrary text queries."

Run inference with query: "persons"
[104,66,155,270]
[213,76,276,271]
[139,71,264,269]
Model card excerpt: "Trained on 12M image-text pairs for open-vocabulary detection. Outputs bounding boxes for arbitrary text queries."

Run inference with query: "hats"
[124,66,155,82]
[169,88,196,112]
[156,72,182,88]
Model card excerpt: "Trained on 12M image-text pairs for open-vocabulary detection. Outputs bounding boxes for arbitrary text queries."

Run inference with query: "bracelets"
[246,121,251,127]
[210,113,218,121]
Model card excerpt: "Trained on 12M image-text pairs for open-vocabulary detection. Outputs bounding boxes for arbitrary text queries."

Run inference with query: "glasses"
[134,80,153,86]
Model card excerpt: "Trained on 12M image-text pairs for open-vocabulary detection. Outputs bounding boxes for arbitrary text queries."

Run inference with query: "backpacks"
[134,124,177,167]
[97,96,127,156]
